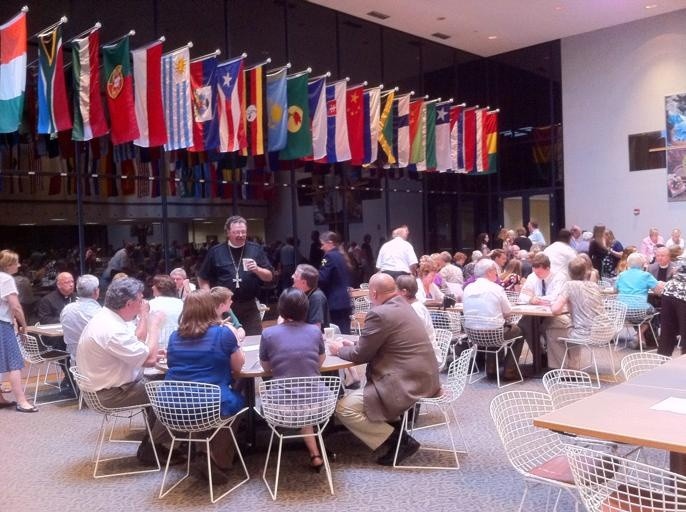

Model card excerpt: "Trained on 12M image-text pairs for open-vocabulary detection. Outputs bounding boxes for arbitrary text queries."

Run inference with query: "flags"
[70,27,110,143]
[100,35,499,177]
[36,22,73,136]
[0,11,27,134]
[530,124,558,180]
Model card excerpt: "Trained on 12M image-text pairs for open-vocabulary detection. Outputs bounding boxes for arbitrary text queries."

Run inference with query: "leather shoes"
[378,436,420,466]
[0,401,16,409]
[136,438,229,485]
[16,402,38,412]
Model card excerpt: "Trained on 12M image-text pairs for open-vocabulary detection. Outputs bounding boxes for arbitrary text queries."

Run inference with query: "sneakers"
[628,335,646,349]
[486,363,521,380]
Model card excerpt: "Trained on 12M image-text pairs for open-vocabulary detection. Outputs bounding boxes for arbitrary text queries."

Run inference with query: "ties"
[542,279,545,296]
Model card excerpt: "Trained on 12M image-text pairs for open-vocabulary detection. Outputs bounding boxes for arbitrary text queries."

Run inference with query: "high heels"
[308,456,323,474]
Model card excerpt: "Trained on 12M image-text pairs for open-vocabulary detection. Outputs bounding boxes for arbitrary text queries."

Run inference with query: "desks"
[17,317,70,341]
[533,353,686,512]
[144,322,372,414]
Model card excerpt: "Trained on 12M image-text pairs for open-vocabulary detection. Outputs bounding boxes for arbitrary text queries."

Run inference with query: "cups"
[242,258,254,272]
[323,327,336,340]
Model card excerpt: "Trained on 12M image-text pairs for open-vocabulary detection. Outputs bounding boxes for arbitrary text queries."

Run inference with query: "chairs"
[621,352,671,381]
[70,365,163,481]
[12,332,77,406]
[344,270,663,388]
[565,443,686,512]
[387,349,472,474]
[143,382,253,505]
[489,392,602,509]
[258,370,344,501]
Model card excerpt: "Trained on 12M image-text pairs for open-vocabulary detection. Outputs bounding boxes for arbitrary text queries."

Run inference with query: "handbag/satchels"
[444,293,456,308]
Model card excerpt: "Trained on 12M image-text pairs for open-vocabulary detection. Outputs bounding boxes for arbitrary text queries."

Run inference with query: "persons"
[15,230,372,323]
[420,220,685,381]
[1,249,38,412]
[197,216,275,336]
[376,224,419,280]
[40,262,442,480]
